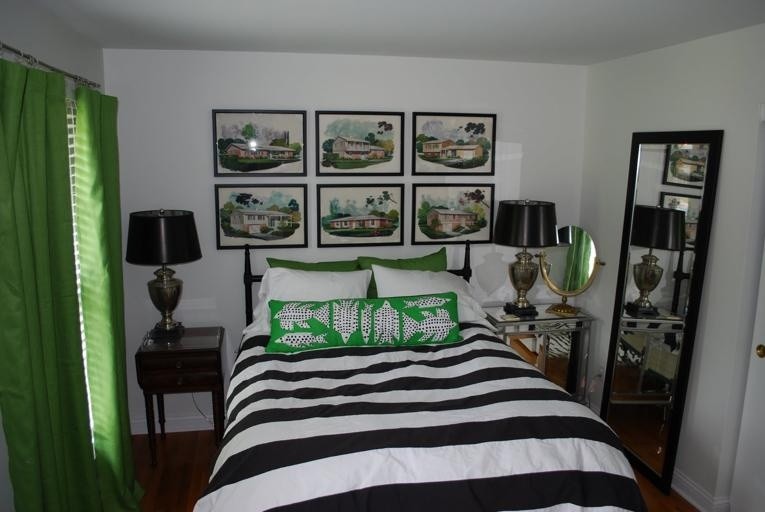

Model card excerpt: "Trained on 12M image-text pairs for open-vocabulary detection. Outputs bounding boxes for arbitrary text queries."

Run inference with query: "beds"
[197,240,658,510]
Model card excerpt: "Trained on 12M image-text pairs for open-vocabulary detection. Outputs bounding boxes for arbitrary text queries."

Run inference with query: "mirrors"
[598,130,727,500]
[543,223,600,318]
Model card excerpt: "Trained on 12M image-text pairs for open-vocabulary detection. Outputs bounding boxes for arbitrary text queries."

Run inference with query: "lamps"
[624,200,688,321]
[491,197,558,317]
[123,207,205,339]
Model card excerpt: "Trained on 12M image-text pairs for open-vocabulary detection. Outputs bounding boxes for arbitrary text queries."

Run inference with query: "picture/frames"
[214,182,309,251]
[411,110,497,177]
[660,141,708,190]
[210,105,308,179]
[410,181,494,248]
[659,189,702,248]
[314,108,407,178]
[315,181,406,248]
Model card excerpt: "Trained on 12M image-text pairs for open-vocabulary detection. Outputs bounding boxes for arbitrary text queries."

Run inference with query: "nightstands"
[483,300,597,404]
[611,310,682,409]
[133,326,229,471]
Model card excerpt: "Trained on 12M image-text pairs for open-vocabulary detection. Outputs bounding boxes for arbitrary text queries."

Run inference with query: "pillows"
[263,255,360,273]
[365,261,487,326]
[353,246,448,302]
[242,267,373,335]
[263,291,466,350]
